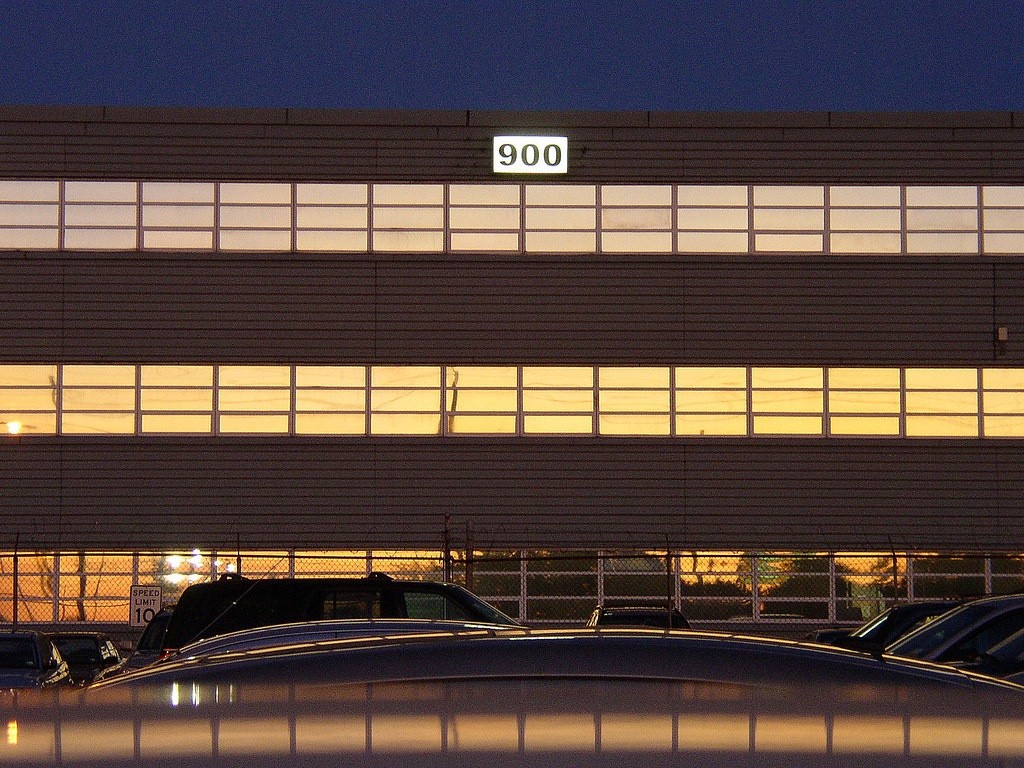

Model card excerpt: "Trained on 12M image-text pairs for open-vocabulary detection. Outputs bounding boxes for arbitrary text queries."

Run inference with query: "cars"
[84,593,1023,713]
[119,602,177,676]
[46,629,123,690]
[1,628,74,691]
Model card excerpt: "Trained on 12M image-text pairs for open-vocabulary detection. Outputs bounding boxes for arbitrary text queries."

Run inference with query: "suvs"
[157,570,523,662]
